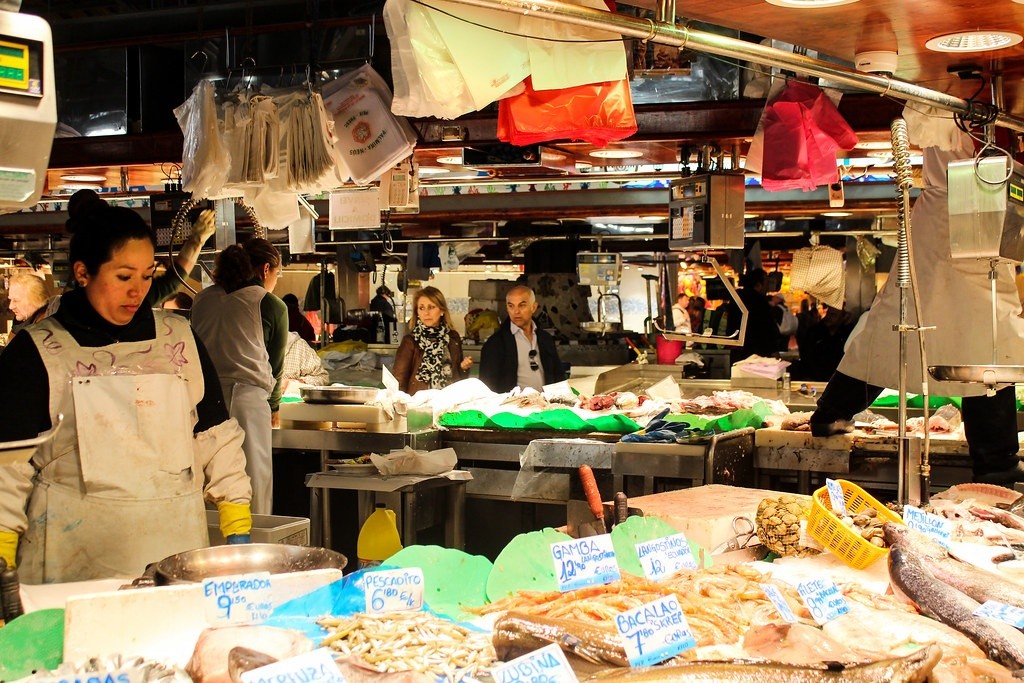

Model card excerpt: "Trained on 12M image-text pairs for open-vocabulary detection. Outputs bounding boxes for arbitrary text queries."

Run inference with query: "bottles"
[782,373,791,390]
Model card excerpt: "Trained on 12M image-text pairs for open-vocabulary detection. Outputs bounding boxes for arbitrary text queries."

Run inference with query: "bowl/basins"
[156,543,348,584]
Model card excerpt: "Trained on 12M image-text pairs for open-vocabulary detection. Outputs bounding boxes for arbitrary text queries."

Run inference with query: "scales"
[651,172,750,347]
[927,155,1024,388]
[577,253,623,335]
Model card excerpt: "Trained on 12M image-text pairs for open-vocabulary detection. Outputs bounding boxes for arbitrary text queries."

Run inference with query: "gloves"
[618,407,692,443]
[177,208,216,266]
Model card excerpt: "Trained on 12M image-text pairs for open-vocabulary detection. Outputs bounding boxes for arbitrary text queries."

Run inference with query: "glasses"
[528,349,539,371]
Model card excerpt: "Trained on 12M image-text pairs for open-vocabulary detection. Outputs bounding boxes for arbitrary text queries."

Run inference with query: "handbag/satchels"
[774,305,799,335]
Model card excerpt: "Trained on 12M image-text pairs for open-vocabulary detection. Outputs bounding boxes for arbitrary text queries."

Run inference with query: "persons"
[162,292,193,308]
[151,210,216,307]
[672,268,820,363]
[282,294,316,341]
[189,239,288,514]
[281,332,329,395]
[0,189,251,579]
[393,286,472,395]
[6,273,49,343]
[481,285,566,393]
[372,286,397,325]
[803,119,1024,490]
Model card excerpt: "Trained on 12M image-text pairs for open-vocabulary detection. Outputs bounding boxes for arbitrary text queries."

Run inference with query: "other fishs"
[881,521,1024,669]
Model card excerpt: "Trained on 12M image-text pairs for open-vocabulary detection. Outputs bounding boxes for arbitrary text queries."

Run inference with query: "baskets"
[806,477,907,572]
[0,608,66,682]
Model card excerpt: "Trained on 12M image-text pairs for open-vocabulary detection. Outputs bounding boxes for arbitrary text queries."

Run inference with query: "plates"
[299,386,380,404]
[326,464,376,474]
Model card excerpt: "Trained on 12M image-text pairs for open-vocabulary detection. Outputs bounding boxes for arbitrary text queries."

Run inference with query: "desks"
[305,469,474,548]
[448,430,982,549]
[267,426,447,539]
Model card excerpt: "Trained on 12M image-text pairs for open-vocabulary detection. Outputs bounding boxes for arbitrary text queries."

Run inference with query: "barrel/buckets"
[654,334,683,365]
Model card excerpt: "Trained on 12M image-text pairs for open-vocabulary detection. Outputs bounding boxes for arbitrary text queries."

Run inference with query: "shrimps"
[460,563,915,668]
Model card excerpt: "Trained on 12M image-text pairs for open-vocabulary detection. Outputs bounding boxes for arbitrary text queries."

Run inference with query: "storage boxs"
[205,509,311,550]
[603,485,813,553]
